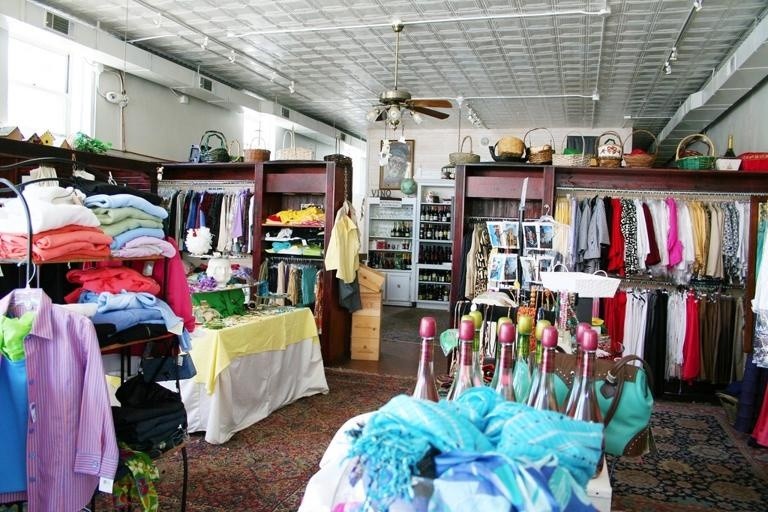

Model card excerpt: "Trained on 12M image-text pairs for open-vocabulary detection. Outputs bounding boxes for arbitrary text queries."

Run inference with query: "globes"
[401,178,417,198]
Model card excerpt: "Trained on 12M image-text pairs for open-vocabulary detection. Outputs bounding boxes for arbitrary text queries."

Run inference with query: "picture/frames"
[379,140,415,190]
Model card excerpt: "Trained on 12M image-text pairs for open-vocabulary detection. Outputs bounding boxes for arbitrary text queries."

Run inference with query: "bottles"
[411,310,558,412]
[391,221,410,237]
[560,321,605,480]
[369,252,411,270]
[725,136,735,158]
[419,204,452,302]
[402,240,409,250]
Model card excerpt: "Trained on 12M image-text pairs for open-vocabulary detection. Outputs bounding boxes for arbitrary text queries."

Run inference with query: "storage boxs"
[350,262,385,361]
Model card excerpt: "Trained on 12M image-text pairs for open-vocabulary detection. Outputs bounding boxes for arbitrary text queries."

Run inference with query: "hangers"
[269,257,311,265]
[625,280,734,296]
[584,187,752,202]
[157,180,254,194]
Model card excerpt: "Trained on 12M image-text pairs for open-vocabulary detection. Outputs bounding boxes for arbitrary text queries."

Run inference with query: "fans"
[368,23,453,120]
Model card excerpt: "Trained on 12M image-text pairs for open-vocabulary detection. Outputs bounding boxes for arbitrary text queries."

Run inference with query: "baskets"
[621,129,658,168]
[551,130,592,167]
[674,133,716,170]
[198,130,231,163]
[595,130,623,167]
[242,149,270,162]
[273,131,317,161]
[522,126,556,165]
[449,136,480,163]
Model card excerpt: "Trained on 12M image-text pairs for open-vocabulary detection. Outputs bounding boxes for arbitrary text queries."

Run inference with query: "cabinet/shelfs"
[0,157,188,512]
[154,160,353,363]
[416,180,456,311]
[365,197,416,307]
[447,162,768,406]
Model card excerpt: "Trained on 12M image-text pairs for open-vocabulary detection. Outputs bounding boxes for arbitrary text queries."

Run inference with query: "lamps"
[664,0,703,74]
[386,107,402,129]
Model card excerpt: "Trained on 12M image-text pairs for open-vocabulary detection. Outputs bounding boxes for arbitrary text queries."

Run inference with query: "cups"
[386,244,400,250]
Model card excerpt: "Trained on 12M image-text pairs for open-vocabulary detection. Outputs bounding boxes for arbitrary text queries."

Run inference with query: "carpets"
[79,366,768,512]
[381,308,450,346]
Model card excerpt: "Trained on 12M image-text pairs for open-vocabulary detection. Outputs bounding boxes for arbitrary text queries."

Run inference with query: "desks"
[157,302,329,446]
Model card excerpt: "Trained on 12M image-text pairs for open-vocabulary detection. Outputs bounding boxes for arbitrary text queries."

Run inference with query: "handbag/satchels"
[596,353,656,458]
[111,339,192,458]
